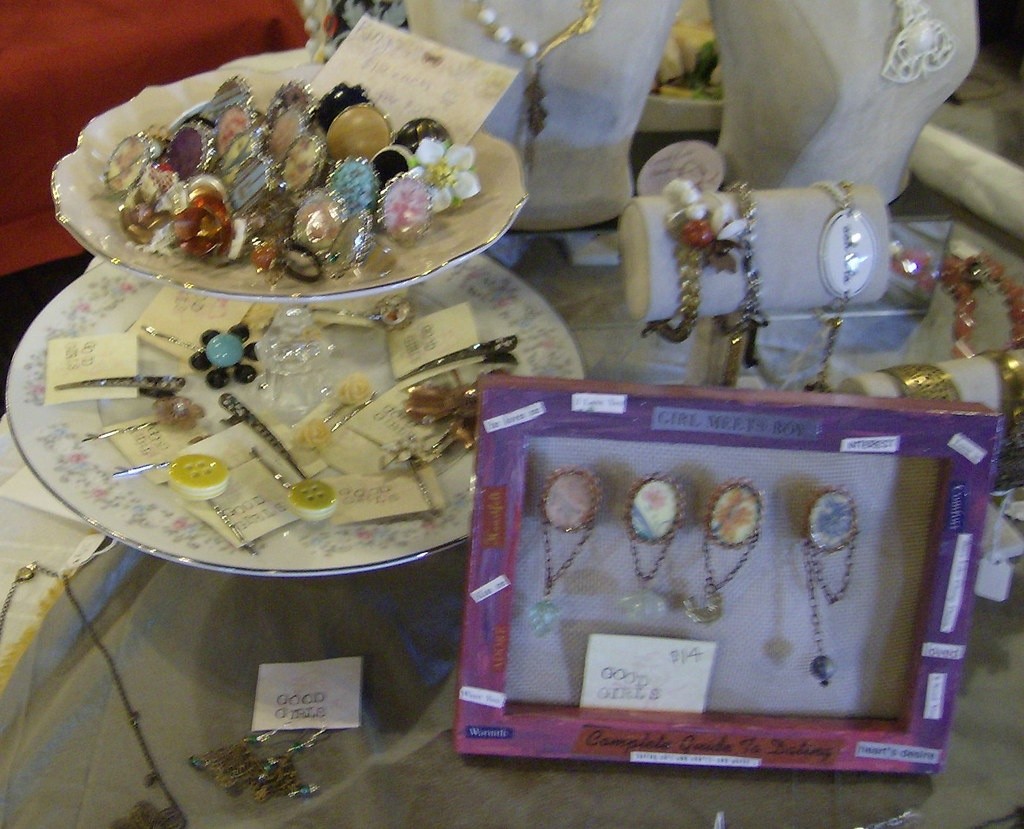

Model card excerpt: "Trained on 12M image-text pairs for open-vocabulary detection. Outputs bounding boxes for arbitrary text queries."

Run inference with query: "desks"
[0,183,1024,829]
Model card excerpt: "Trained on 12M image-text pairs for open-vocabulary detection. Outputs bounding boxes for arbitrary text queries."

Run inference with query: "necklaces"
[478,0,603,136]
[880,0,955,84]
[682,481,764,624]
[0,559,191,829]
[943,253,1024,360]
[802,488,860,687]
[618,470,689,620]
[527,466,603,639]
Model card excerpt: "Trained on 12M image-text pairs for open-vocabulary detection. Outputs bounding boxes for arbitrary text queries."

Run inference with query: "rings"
[103,73,481,285]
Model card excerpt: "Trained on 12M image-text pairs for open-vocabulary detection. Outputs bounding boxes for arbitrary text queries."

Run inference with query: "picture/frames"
[453,374,1001,775]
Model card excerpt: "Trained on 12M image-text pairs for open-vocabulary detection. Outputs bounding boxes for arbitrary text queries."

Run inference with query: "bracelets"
[875,351,1024,491]
[636,176,866,394]
